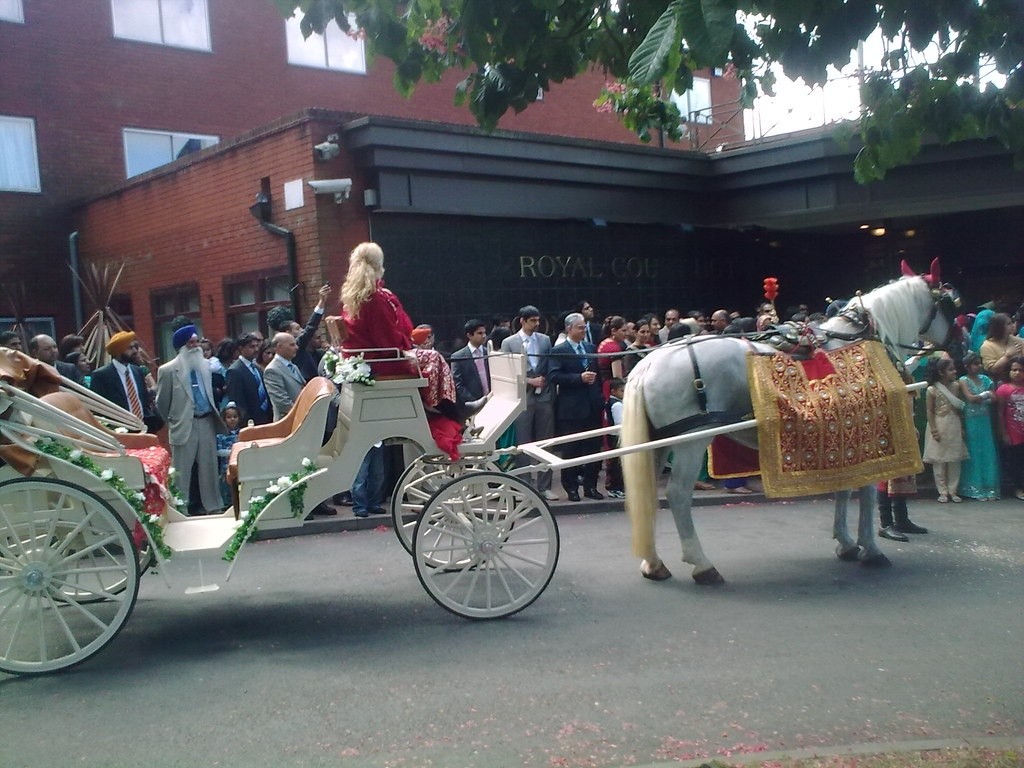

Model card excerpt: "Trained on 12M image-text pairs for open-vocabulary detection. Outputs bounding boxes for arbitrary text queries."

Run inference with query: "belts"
[194,412,214,419]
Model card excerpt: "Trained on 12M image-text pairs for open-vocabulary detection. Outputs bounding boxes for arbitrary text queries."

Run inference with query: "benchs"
[34,391,172,516]
[227,375,338,519]
[325,316,424,382]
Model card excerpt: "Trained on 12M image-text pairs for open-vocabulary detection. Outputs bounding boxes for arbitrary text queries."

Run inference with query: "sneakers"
[313,504,337,516]
[304,514,314,520]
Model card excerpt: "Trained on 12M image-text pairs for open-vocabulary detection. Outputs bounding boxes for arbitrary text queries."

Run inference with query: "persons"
[341,242,488,425]
[0,286,1024,540]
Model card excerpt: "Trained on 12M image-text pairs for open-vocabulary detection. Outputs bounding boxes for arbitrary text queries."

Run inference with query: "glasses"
[582,304,594,312]
[475,331,486,335]
[765,309,771,313]
[127,343,141,350]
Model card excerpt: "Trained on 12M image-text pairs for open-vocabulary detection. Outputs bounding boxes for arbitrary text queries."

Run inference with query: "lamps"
[678,224,695,232]
[588,218,610,231]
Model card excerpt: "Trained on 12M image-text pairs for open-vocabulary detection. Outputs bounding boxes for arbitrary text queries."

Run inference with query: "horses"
[619,256,957,585]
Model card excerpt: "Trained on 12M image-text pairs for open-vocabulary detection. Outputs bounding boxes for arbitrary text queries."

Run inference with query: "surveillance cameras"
[314,141,339,160]
[308,178,352,194]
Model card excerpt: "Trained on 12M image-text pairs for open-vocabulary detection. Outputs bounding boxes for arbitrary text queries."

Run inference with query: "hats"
[173,325,197,354]
[105,331,138,358]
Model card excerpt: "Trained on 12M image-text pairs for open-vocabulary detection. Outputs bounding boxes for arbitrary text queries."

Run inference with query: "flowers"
[34,422,186,562]
[222,458,320,562]
[324,351,376,387]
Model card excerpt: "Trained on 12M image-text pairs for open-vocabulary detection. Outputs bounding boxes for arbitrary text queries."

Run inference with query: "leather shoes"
[879,525,909,541]
[897,518,928,535]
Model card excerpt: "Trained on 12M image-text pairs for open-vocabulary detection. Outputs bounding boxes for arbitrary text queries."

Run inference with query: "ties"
[251,364,269,412]
[190,370,210,414]
[474,349,489,396]
[585,323,592,344]
[577,345,589,372]
[124,369,143,422]
[287,364,305,387]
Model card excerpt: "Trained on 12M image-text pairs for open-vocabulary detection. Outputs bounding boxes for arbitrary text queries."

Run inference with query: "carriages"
[0,239,976,675]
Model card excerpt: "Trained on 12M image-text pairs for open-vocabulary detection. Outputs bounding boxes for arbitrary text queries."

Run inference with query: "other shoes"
[355,511,369,517]
[369,508,387,514]
[513,480,754,511]
[333,497,354,506]
[936,487,1024,503]
